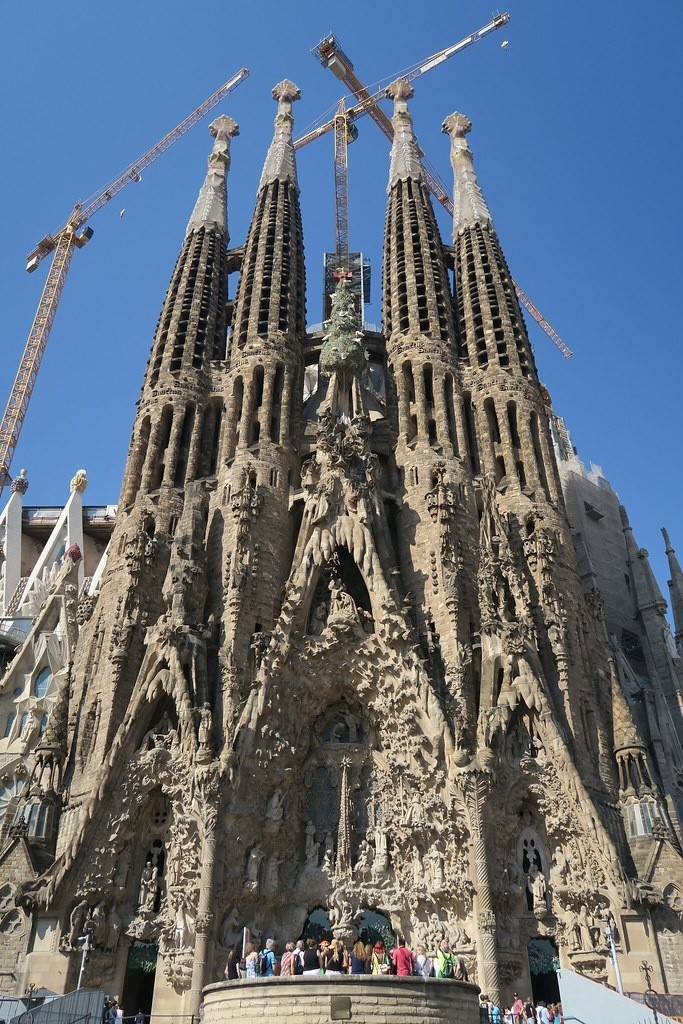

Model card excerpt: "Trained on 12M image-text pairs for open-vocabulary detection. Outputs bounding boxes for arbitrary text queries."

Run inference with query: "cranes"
[0,64,257,493]
[290,11,513,318]
[307,30,577,360]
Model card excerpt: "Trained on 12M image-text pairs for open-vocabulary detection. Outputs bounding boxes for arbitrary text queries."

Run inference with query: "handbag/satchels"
[413,970,422,976]
[239,958,247,971]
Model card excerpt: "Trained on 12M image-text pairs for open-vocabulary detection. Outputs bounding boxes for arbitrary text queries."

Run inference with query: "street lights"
[638,960,662,1024]
[17,983,40,1024]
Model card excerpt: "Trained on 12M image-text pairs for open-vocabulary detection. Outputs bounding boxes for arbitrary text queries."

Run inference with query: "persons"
[479,992,564,1024]
[300,407,381,525]
[194,702,213,749]
[428,844,443,884]
[528,865,546,901]
[310,602,327,636]
[562,904,595,951]
[100,1000,124,1024]
[357,607,375,634]
[328,578,347,602]
[410,846,420,860]
[550,846,566,879]
[152,711,173,735]
[533,736,542,758]
[416,912,468,952]
[352,840,373,873]
[304,821,334,877]
[134,1009,144,1024]
[138,862,158,904]
[69,900,87,948]
[224,936,466,982]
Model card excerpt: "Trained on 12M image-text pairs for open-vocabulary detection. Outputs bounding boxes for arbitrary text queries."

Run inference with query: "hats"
[319,941,329,946]
[513,992,518,996]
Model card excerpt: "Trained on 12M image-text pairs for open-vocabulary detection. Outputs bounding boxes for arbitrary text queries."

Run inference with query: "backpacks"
[258,950,273,974]
[294,951,304,974]
[375,953,388,975]
[440,951,454,978]
